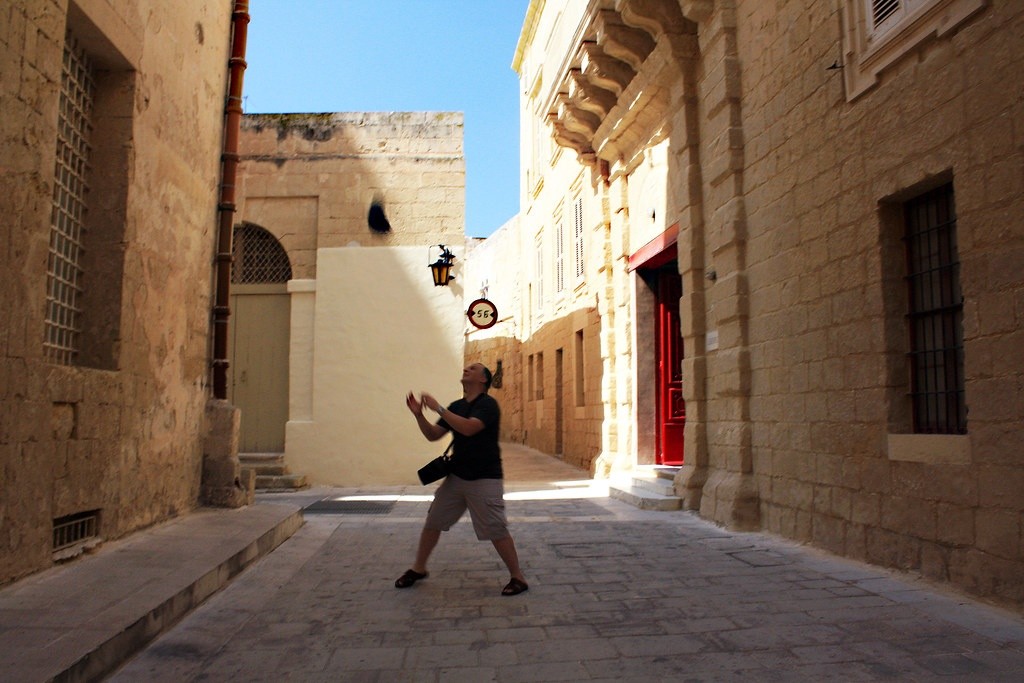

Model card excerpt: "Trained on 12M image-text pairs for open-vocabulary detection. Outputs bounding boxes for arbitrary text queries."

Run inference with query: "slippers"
[395,568,430,588]
[502,577,528,595]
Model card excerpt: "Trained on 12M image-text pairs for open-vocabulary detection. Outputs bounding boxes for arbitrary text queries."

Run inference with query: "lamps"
[428,243,456,286]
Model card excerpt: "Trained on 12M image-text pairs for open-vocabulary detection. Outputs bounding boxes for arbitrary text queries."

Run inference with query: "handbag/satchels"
[417,456,449,486]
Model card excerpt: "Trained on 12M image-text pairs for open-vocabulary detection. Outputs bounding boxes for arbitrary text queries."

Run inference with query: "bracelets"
[437,406,444,414]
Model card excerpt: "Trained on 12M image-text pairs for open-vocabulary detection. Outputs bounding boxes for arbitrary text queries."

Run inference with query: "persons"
[395,363,529,597]
